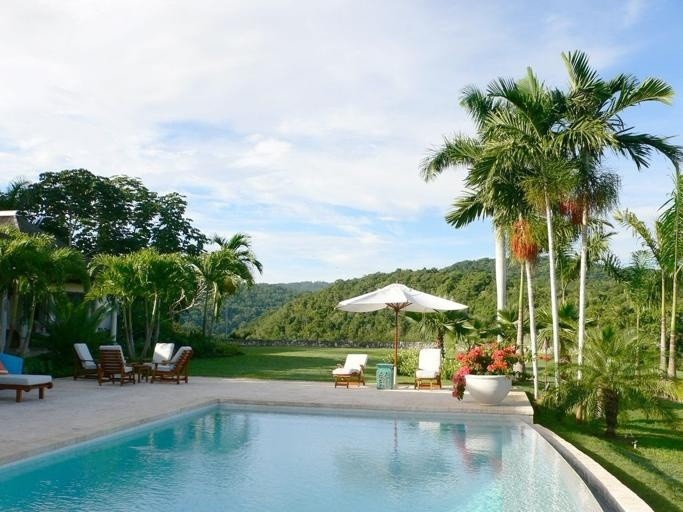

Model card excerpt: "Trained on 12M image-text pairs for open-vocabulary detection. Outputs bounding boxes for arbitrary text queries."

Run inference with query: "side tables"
[375,363,395,389]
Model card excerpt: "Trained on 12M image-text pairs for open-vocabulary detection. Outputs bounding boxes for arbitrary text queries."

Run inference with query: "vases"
[463,375,513,405]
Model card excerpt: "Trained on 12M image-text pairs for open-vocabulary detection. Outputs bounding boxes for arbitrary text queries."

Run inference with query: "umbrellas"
[333,280,469,389]
[336,415,466,502]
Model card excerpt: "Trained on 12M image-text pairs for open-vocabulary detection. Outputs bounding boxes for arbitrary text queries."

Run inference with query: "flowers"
[452,343,526,400]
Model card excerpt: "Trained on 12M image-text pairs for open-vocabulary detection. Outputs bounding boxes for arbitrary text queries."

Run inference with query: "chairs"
[73,338,190,386]
[416,347,443,390]
[331,352,370,390]
[0,354,53,404]
